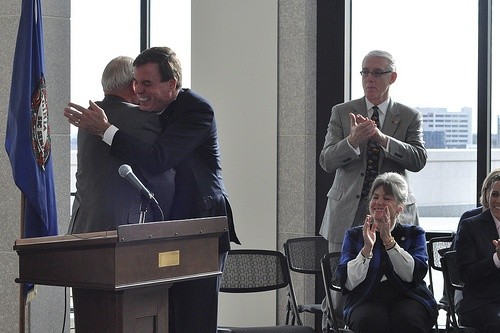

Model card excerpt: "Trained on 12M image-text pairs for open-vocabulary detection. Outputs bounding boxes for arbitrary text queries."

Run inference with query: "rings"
[75,118,80,123]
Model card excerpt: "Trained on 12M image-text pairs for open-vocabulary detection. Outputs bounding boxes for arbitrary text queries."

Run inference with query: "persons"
[64,46,241,333]
[319,51,428,333]
[336,173,439,333]
[452,170,500,333]
[66,56,175,235]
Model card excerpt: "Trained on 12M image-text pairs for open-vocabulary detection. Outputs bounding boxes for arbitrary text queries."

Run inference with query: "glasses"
[360,69,393,77]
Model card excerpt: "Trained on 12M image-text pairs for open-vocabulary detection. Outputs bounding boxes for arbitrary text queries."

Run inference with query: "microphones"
[118,164,159,207]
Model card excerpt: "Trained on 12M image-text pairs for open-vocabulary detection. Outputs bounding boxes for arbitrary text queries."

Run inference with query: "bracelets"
[383,238,394,246]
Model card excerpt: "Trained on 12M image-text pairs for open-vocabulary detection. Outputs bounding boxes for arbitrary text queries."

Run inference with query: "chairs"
[216,237,353,333]
[425,232,486,333]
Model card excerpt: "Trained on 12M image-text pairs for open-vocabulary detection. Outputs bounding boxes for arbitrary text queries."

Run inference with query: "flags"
[5,0,58,305]
